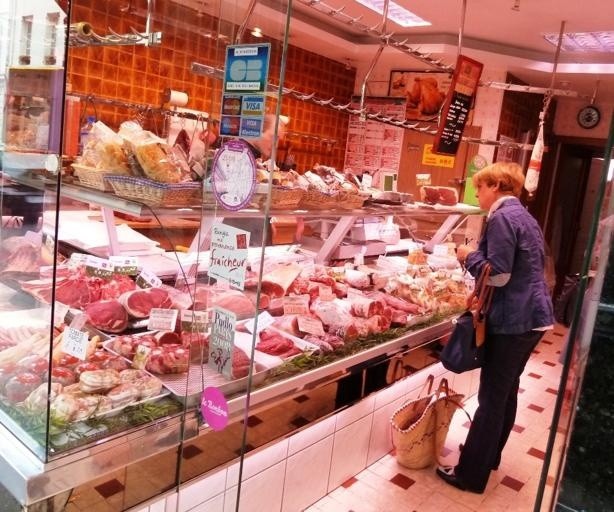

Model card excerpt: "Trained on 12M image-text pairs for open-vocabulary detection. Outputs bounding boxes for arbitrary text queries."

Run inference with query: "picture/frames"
[386,68,474,127]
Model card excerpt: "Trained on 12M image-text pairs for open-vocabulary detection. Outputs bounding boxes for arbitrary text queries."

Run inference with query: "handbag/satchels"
[439,310,485,373]
[389,375,464,470]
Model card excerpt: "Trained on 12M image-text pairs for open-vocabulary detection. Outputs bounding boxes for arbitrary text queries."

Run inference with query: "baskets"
[201,177,368,212]
[73,164,126,192]
[108,173,200,206]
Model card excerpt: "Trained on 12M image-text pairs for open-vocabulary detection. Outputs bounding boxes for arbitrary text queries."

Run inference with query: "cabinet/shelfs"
[0,172,486,512]
[548,224,613,510]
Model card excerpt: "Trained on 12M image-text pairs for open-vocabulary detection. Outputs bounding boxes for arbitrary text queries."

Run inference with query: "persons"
[435,161,555,495]
[223,115,287,247]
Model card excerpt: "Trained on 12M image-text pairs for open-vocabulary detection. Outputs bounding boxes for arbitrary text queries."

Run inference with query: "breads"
[98,144,182,183]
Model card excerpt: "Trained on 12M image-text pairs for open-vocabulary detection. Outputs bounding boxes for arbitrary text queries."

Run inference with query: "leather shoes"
[436,466,483,492]
[457,443,499,469]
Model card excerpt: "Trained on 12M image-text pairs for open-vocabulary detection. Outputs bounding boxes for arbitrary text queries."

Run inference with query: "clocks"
[577,106,600,129]
